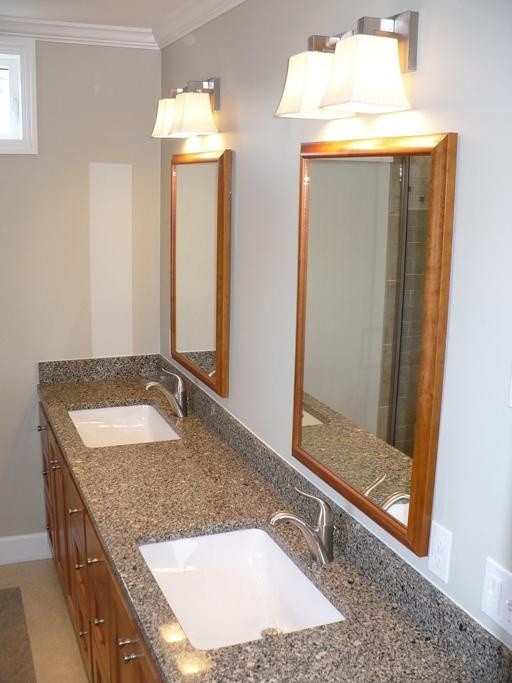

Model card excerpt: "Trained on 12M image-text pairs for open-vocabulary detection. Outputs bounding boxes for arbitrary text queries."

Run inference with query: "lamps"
[151,77,220,138]
[275,10,419,120]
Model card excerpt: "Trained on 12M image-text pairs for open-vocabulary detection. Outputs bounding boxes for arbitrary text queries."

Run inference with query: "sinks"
[302,407,323,426]
[67,404,182,448]
[139,528,346,650]
[388,504,410,528]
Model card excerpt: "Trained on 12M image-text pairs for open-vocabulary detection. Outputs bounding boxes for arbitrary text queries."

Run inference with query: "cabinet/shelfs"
[38,402,164,683]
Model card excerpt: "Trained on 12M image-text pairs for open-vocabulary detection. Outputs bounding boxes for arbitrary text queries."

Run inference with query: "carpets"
[0,586,37,683]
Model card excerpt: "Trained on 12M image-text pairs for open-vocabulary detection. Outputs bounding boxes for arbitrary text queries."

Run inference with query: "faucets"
[207,369,216,378]
[362,471,410,515]
[145,368,187,417]
[267,486,334,563]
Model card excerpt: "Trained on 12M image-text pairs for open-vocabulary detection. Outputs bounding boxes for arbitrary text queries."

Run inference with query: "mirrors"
[171,150,233,398]
[292,132,459,557]
